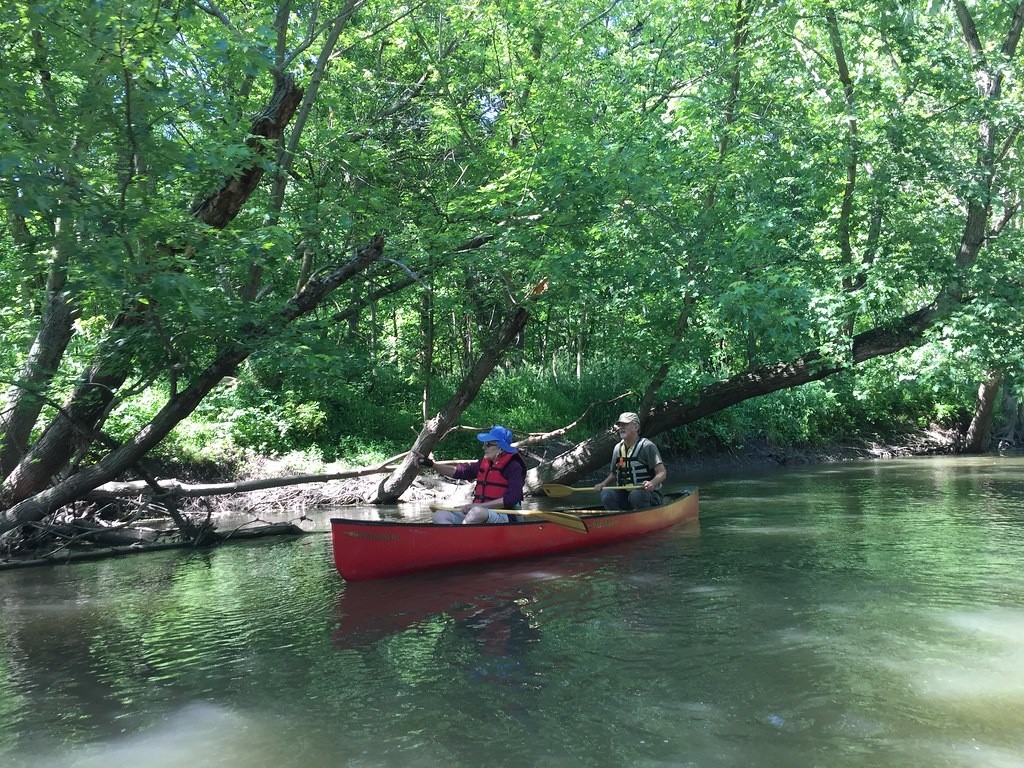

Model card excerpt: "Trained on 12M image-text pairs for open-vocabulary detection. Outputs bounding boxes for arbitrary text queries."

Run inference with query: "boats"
[329,484,700,582]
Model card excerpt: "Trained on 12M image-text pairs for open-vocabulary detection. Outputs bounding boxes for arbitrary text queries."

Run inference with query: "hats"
[616,412,640,425]
[477,426,516,453]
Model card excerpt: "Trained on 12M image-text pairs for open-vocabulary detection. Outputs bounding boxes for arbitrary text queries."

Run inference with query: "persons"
[412,425,528,523]
[593,412,666,510]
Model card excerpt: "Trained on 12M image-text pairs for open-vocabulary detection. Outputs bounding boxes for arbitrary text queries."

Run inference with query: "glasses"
[484,441,498,448]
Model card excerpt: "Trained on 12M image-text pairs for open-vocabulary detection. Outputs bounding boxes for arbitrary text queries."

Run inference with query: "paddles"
[538,481,654,499]
[427,501,591,534]
